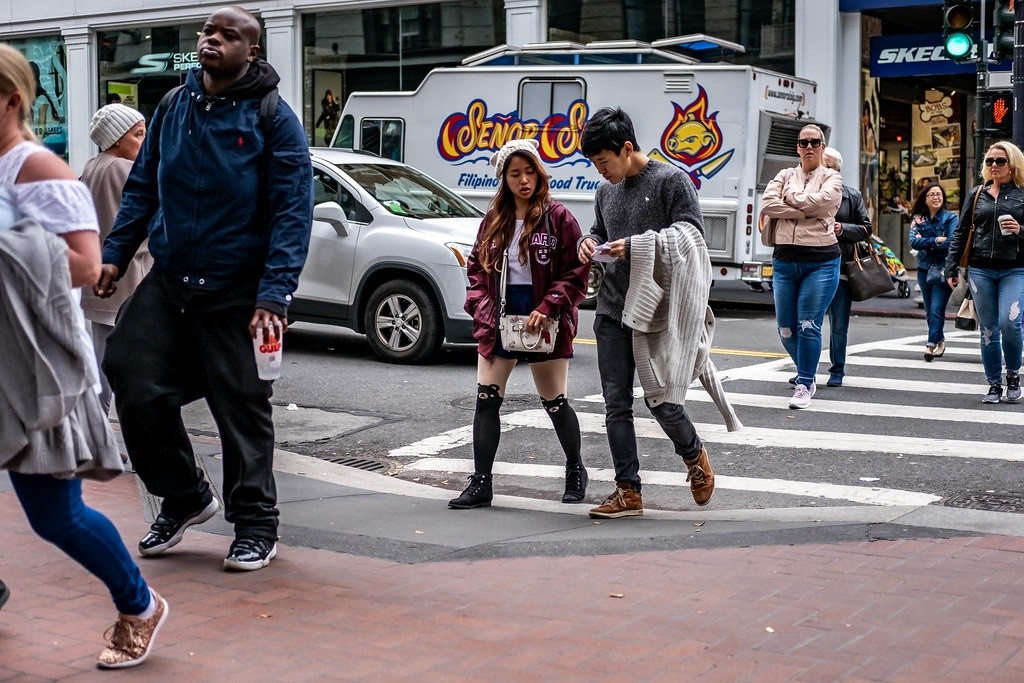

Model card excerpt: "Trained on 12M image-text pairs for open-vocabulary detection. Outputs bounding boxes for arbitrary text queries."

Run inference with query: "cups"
[997,214,1012,235]
[253,320,281,380]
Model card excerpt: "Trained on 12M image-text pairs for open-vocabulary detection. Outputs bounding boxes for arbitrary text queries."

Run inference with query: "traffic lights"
[993,0,1015,59]
[942,0,975,61]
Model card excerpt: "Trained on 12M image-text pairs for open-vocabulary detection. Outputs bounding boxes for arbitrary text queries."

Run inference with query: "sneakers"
[561,456,589,503]
[97,587,169,668]
[1005,374,1022,401]
[981,384,1004,403]
[682,443,716,505]
[448,472,493,508]
[589,482,643,518]
[0,578,10,608]
[222,537,279,570]
[790,381,816,409]
[138,494,220,556]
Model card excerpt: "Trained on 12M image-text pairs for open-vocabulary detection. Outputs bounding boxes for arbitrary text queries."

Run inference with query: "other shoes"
[120,453,128,464]
[933,346,946,356]
[924,352,934,362]
[790,373,799,384]
[826,370,845,386]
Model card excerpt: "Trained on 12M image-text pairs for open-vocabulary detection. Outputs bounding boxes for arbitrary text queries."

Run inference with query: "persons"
[945,141,1024,406]
[315,85,341,147]
[446,139,590,509]
[98,5,314,569]
[761,124,844,408]
[76,101,154,426]
[822,143,872,388]
[577,107,716,520]
[909,180,959,362]
[0,40,169,669]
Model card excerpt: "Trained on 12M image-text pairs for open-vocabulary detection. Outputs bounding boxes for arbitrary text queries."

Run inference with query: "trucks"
[319,30,834,311]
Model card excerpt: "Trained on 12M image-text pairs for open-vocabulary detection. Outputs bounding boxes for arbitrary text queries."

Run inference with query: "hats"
[90,102,145,152]
[490,139,544,178]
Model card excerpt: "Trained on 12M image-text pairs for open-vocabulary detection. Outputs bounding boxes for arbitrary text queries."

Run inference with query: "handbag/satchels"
[959,184,984,268]
[926,264,948,283]
[500,312,559,354]
[954,287,978,331]
[842,226,896,301]
[761,167,794,247]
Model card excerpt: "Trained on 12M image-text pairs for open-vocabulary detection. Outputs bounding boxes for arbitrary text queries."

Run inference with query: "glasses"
[984,157,1009,167]
[926,193,943,200]
[797,139,822,149]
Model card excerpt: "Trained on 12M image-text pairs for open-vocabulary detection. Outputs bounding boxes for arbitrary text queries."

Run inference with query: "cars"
[288,146,488,366]
